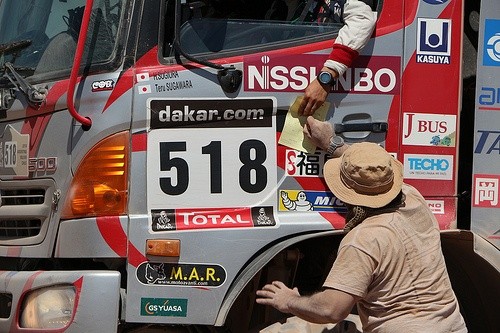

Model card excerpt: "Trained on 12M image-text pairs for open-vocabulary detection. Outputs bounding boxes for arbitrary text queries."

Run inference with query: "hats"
[323,141,404,208]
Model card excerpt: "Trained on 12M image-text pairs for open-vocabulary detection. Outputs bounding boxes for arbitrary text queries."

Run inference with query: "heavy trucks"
[0,0,500,333]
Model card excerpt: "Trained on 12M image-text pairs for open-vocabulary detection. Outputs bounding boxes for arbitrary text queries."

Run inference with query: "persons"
[257,116,469,333]
[261,0,378,116]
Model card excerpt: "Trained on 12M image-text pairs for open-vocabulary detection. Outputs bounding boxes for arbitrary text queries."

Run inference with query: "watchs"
[327,135,344,155]
[317,71,335,88]
